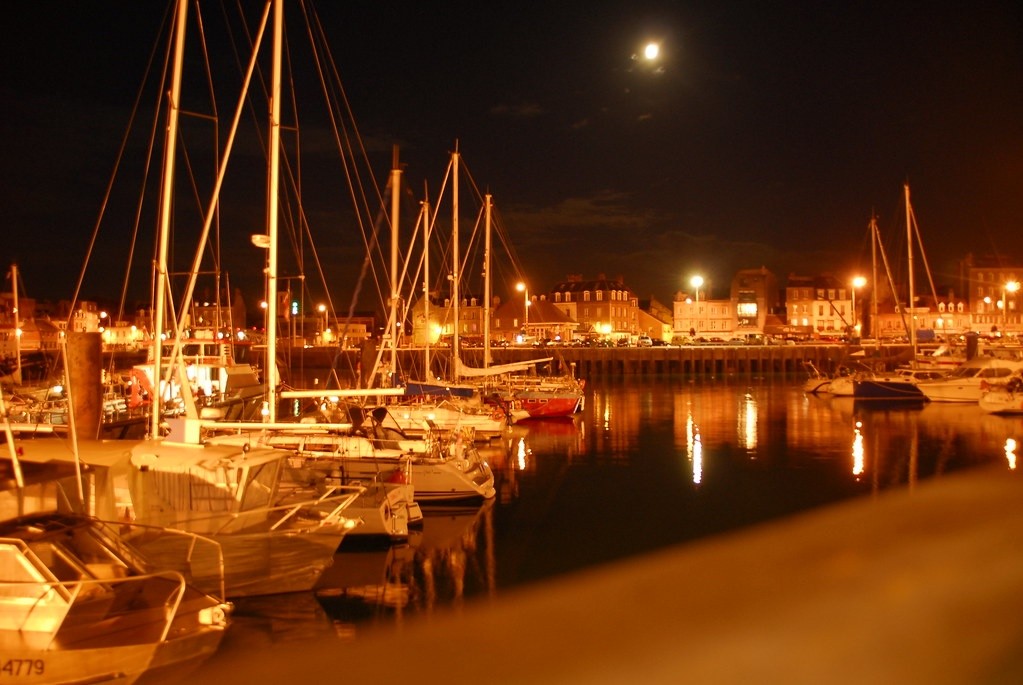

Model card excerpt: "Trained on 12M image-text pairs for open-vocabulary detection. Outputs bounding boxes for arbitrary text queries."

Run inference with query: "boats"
[979,371,1023,414]
[916,359,1023,402]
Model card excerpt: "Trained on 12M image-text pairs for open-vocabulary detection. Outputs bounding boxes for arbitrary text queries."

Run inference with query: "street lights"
[852,277,864,325]
[1002,283,1017,322]
[101,313,112,344]
[517,283,528,335]
[319,306,328,328]
[693,277,700,335]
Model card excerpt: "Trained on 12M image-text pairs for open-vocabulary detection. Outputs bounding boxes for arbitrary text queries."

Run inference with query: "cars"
[651,338,665,346]
[637,336,652,347]
[959,332,991,340]
[729,338,746,345]
[531,338,598,348]
[597,339,615,348]
[710,337,729,346]
[672,336,695,346]
[693,337,710,342]
[448,339,509,348]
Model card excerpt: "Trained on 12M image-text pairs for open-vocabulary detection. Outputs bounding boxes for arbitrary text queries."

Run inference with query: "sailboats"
[801,185,984,397]
[0,0,587,684]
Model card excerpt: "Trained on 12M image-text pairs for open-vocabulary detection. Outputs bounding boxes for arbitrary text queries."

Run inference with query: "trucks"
[903,330,934,343]
